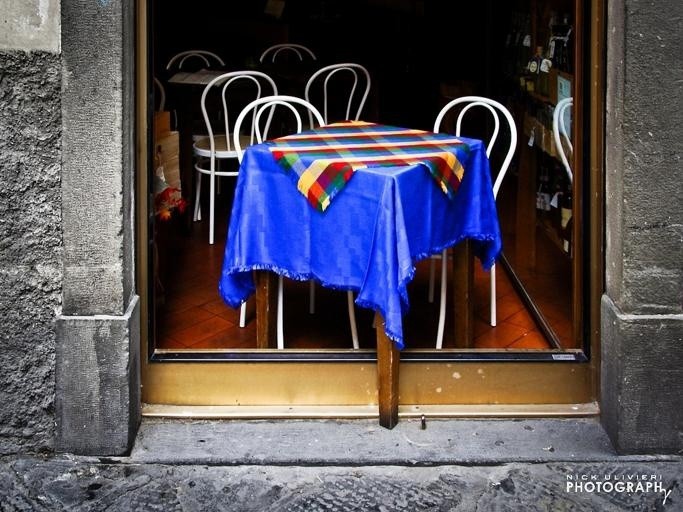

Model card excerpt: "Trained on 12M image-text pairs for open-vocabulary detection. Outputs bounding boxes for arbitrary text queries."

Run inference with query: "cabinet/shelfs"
[522,0,575,269]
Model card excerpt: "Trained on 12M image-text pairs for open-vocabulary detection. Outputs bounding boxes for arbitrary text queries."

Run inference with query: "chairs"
[230,92,361,353]
[550,92,576,185]
[149,74,171,114]
[192,69,277,245]
[259,43,318,66]
[164,49,225,71]
[420,95,518,351]
[305,62,370,124]
